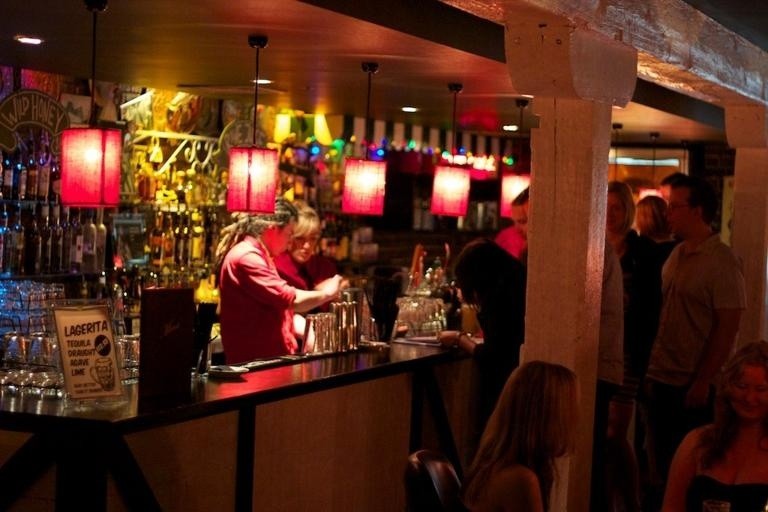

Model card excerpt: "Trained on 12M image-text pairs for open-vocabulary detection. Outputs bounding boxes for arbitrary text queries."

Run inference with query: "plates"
[206,363,251,377]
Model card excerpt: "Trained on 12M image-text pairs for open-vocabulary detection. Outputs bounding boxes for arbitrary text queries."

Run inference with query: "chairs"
[404,447,464,511]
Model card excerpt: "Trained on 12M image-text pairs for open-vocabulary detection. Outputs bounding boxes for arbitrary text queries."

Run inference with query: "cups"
[299,314,338,357]
[0,327,140,397]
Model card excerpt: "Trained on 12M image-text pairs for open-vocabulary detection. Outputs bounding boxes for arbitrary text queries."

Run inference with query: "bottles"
[130,87,152,128]
[1,152,227,325]
[315,214,378,261]
[401,256,477,340]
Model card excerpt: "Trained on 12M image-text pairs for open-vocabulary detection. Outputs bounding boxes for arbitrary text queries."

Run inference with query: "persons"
[272,198,352,354]
[438,171,768,512]
[212,196,346,365]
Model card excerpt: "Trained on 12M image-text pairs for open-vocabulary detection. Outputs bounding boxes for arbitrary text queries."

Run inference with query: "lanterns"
[429,165,472,217]
[342,157,388,216]
[61,129,123,209]
[227,147,280,214]
